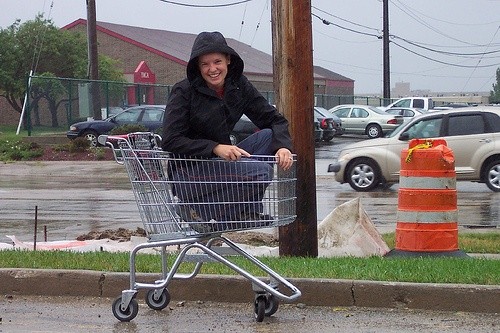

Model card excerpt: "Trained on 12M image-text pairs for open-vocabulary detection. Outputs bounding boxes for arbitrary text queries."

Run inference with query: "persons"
[161,30,293,234]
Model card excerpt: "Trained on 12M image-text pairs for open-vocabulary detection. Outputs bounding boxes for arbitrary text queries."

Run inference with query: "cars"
[328,104,403,139]
[383,108,424,122]
[229,115,259,145]
[67,105,166,147]
[313,110,336,141]
[328,106,500,192]
[314,116,323,147]
[313,106,346,137]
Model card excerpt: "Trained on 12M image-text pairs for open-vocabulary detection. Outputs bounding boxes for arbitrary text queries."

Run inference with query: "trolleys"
[96,131,302,322]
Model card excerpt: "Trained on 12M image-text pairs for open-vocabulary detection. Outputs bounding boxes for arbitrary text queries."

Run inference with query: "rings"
[288,157,293,161]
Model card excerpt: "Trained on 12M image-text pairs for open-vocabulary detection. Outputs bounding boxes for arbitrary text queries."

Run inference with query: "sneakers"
[228,212,274,227]
[173,200,210,233]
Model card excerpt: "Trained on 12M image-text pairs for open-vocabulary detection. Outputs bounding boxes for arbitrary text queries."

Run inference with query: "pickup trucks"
[377,96,453,112]
